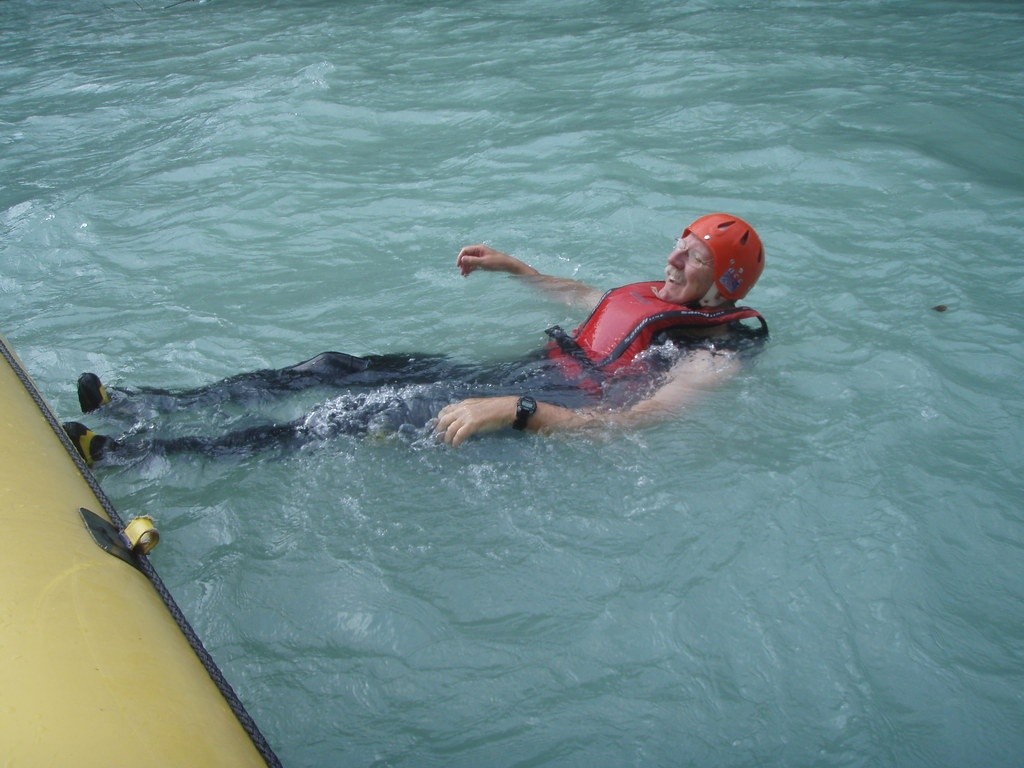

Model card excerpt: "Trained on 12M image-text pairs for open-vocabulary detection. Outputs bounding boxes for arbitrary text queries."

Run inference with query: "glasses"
[673,237,713,268]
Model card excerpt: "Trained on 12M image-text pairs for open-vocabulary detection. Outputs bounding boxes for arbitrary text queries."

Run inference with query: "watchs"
[512,395,537,432]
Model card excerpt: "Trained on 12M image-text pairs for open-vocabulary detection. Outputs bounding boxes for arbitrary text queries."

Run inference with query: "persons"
[61,208,773,472]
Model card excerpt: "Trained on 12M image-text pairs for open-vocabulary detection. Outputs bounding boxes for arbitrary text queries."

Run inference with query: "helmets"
[682,213,764,300]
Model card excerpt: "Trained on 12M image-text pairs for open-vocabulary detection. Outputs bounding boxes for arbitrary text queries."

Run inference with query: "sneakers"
[77,373,109,414]
[62,421,108,469]
